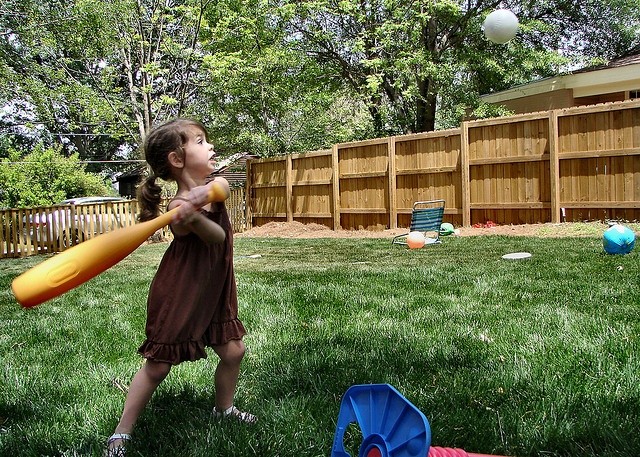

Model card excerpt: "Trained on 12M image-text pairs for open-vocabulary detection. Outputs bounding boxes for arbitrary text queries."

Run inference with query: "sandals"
[211,406,258,426]
[104,434,132,456]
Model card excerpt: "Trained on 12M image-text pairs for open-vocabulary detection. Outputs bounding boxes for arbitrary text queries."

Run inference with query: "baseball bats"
[11,176,228,308]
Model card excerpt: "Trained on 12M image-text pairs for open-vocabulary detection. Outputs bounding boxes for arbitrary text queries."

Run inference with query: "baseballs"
[407,231,425,249]
[483,8,521,44]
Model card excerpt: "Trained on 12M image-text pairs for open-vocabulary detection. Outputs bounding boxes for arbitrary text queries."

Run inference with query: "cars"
[23,196,139,251]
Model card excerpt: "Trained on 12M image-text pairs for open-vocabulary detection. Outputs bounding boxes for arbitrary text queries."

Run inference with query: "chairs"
[391,199,445,246]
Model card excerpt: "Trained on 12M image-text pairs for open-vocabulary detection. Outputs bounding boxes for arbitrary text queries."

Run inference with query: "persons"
[101,116,259,456]
